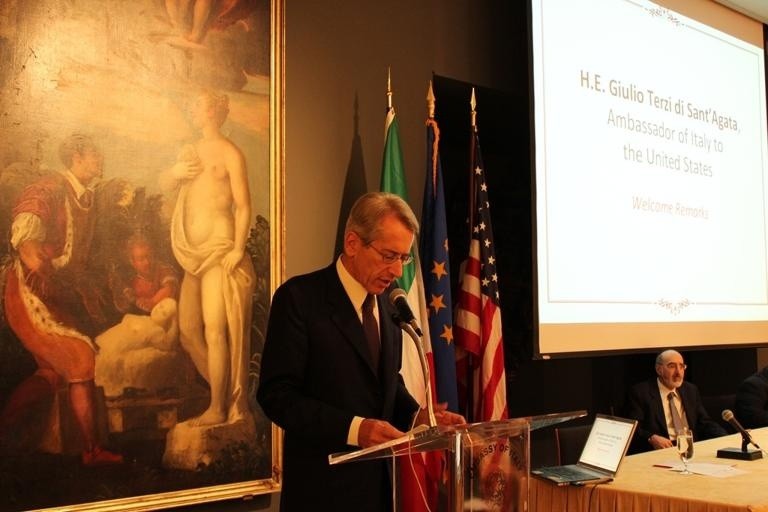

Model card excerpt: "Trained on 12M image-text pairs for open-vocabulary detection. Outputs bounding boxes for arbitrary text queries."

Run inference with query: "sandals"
[82,447,123,466]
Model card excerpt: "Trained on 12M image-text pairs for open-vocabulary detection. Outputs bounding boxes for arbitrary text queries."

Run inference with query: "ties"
[667,392,683,433]
[361,292,380,368]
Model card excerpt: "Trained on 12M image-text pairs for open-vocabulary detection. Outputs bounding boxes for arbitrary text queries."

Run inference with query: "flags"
[378,68,442,511]
[453,87,511,511]
[418,78,461,417]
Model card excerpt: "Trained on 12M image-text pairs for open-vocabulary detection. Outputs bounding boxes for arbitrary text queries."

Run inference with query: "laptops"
[531,413,638,488]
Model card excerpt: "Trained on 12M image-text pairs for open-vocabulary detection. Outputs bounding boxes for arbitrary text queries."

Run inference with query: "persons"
[126,234,177,311]
[160,92,251,426]
[0,134,126,467]
[95,295,177,353]
[629,348,729,454]
[255,191,467,512]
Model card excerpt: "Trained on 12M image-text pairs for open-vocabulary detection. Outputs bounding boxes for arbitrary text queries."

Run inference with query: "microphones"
[389,288,419,334]
[721,409,761,450]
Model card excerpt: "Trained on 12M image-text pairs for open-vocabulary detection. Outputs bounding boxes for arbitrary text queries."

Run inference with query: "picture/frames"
[1,2,285,512]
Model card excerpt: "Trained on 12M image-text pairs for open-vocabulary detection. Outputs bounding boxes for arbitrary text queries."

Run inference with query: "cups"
[677,430,694,475]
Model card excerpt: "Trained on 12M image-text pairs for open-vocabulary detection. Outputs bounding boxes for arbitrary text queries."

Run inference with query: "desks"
[518,425,767,512]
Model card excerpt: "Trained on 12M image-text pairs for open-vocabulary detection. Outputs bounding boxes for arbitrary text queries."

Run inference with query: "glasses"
[667,363,687,370]
[354,231,414,266]
